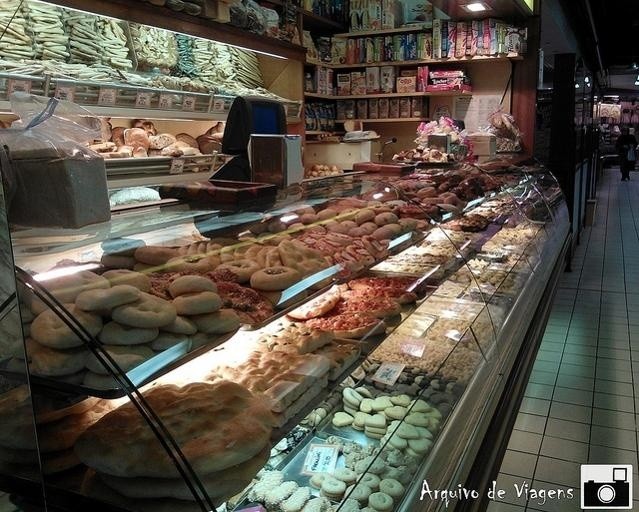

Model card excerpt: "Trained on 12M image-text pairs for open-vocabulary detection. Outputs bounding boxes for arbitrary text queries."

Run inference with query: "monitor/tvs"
[208,96,287,181]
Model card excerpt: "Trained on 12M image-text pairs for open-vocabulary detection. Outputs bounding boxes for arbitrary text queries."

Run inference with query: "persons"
[615,126,637,181]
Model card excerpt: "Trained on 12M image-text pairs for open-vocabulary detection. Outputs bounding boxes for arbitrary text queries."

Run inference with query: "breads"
[0,113,561,512]
